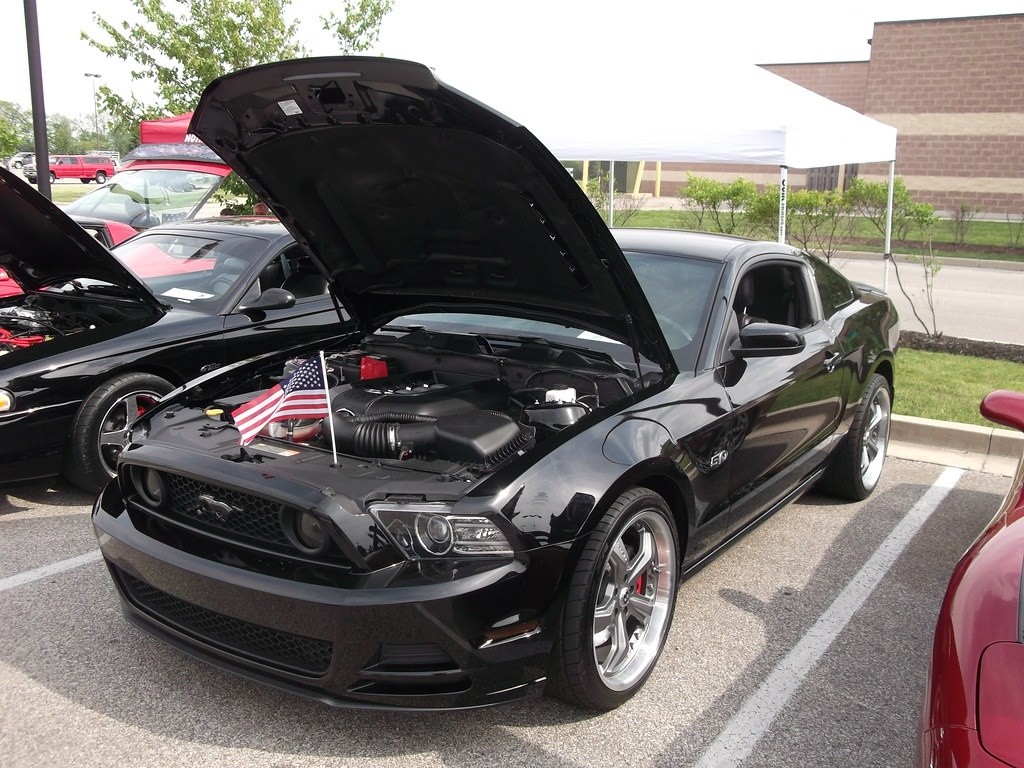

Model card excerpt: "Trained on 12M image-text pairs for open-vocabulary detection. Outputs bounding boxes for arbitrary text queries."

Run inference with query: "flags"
[230,354,330,447]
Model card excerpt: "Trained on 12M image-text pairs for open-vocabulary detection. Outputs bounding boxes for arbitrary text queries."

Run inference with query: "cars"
[921,389,1024,767]
[0,52,902,712]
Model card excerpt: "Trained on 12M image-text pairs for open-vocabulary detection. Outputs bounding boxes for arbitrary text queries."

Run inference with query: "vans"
[8,151,34,169]
[22,154,115,184]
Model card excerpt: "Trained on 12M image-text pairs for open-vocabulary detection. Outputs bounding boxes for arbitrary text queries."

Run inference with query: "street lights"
[83,72,102,152]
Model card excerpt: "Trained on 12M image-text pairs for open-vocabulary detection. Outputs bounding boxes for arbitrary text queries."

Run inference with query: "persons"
[220,208,238,216]
[253,203,268,215]
